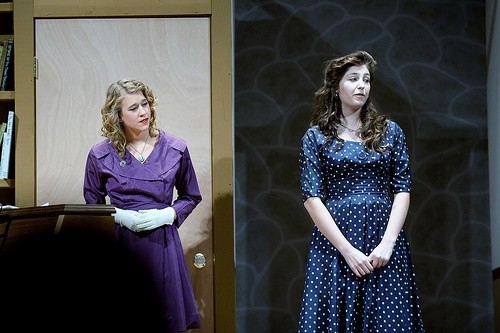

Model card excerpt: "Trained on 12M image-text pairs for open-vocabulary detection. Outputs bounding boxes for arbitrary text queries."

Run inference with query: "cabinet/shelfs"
[0,0,15,188]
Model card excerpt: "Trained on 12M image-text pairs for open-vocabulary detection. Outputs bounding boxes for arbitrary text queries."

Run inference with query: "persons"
[83,80,204,333]
[296,52,428,333]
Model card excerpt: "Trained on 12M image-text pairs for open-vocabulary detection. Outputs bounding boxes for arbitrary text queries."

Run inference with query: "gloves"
[111,206,140,232]
[135,207,175,230]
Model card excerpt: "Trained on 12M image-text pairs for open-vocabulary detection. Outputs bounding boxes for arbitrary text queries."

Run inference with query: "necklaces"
[126,132,150,162]
[338,120,364,138]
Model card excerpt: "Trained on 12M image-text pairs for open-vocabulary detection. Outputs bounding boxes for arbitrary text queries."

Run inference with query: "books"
[0,111,15,180]
[0,38,14,91]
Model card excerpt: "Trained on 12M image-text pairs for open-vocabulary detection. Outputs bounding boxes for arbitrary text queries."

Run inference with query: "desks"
[0,203,116,245]
